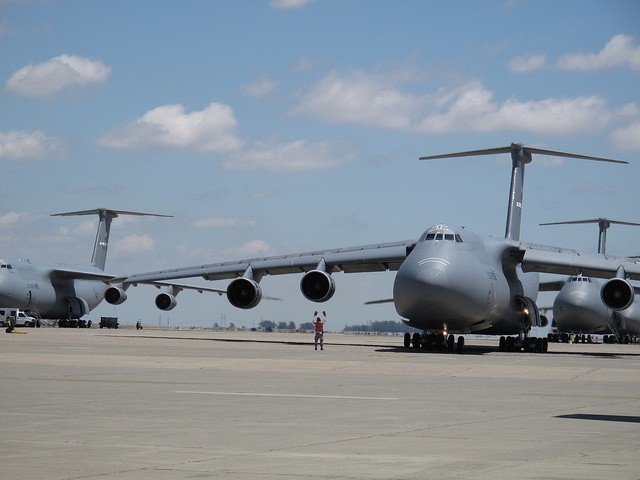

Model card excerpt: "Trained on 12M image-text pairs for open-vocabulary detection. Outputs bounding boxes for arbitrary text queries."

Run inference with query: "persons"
[311,310,327,350]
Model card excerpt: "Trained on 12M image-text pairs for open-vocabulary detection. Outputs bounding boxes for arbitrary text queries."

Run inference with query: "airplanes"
[123,141,639,354]
[1,208,282,328]
[365,218,638,351]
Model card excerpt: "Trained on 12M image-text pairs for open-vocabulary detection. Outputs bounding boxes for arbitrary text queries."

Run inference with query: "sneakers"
[314,347,317,350]
[320,347,324,350]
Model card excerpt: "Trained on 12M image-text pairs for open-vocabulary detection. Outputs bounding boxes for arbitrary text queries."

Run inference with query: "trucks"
[1,307,36,327]
